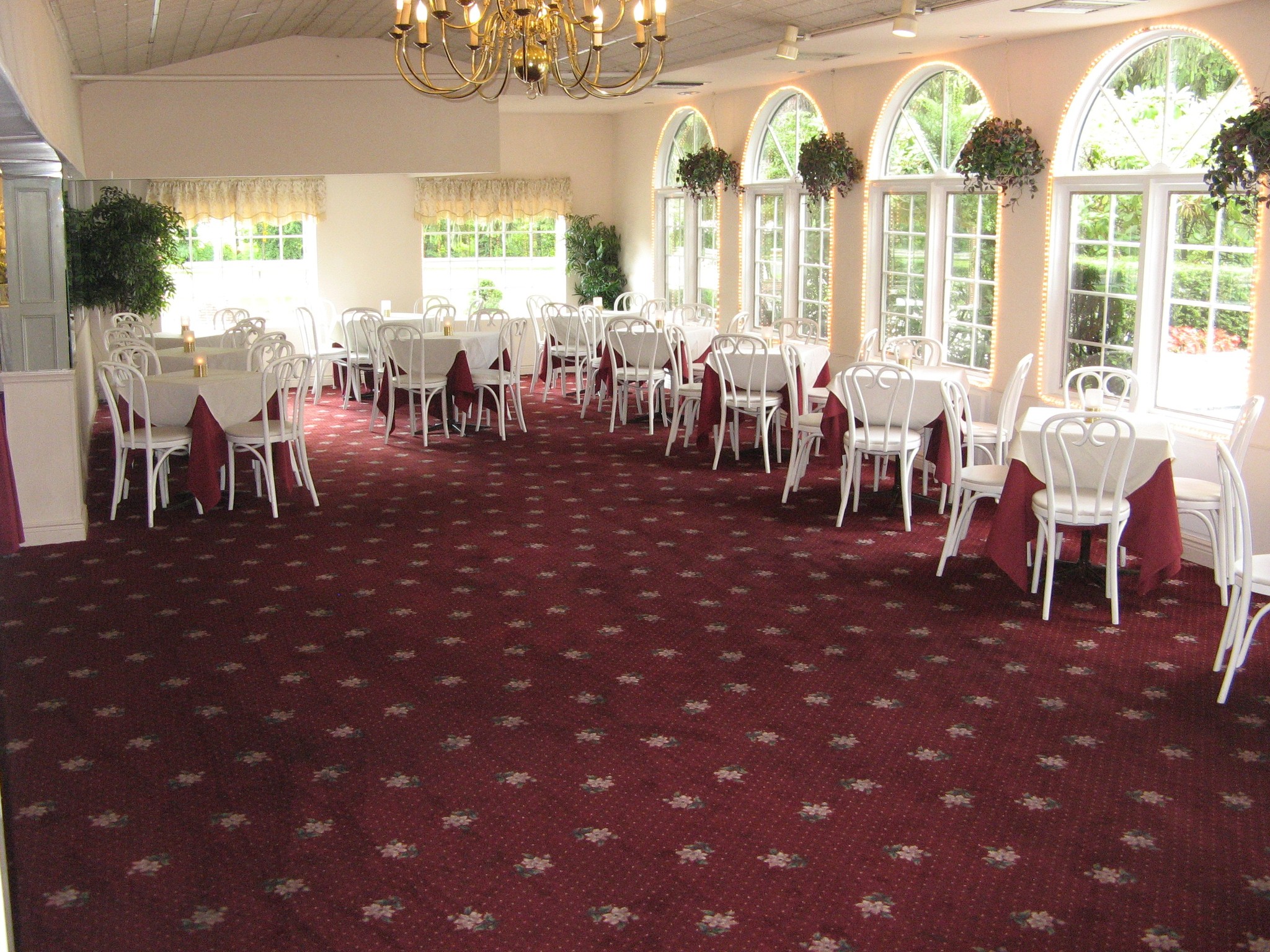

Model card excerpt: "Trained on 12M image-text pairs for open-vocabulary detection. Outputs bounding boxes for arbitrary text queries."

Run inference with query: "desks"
[332,312,441,399]
[694,345,833,457]
[377,328,512,437]
[144,345,259,374]
[599,322,719,424]
[115,366,296,515]
[139,327,245,348]
[981,404,1184,599]
[821,366,968,511]
[538,310,645,396]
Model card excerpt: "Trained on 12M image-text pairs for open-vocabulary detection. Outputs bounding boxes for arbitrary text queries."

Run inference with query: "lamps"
[892,0,931,39]
[388,0,669,101]
[776,24,811,61]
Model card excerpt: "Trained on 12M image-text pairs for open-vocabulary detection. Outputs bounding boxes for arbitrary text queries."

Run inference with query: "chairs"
[322,290,527,447]
[89,307,320,528]
[527,291,1270,705]
